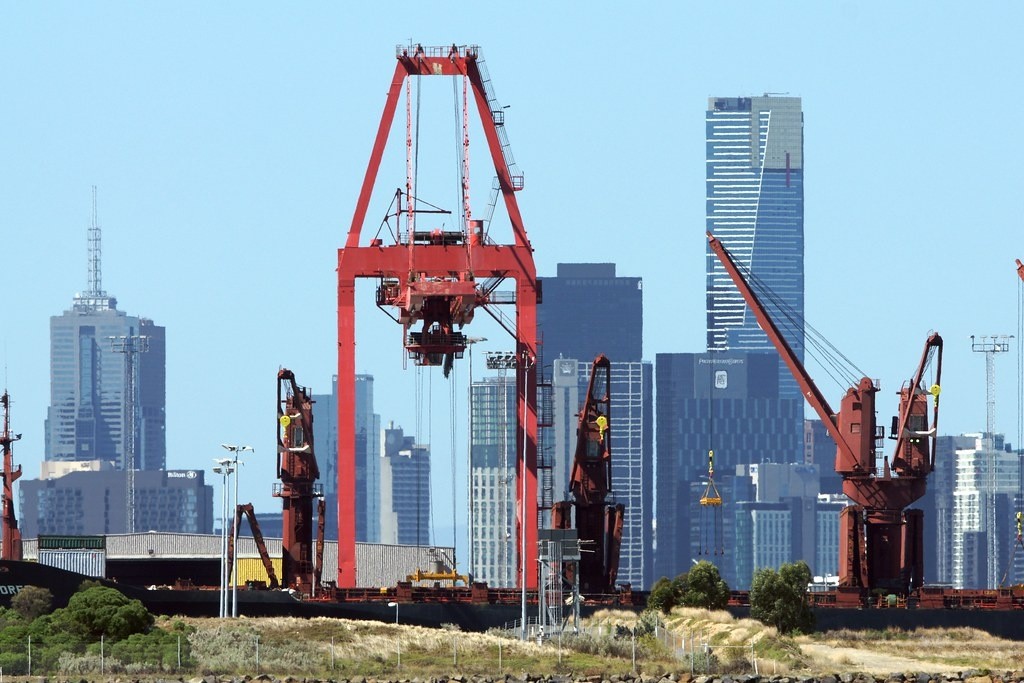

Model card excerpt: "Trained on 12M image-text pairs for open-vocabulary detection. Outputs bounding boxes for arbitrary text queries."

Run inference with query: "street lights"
[213,458,244,619]
[222,445,255,619]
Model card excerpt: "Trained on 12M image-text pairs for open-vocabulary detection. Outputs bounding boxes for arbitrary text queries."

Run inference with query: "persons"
[443,324,450,344]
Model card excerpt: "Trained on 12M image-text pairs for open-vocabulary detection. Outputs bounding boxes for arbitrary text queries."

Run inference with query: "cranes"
[699,231,944,607]
[552,356,625,600]
[272,365,321,593]
